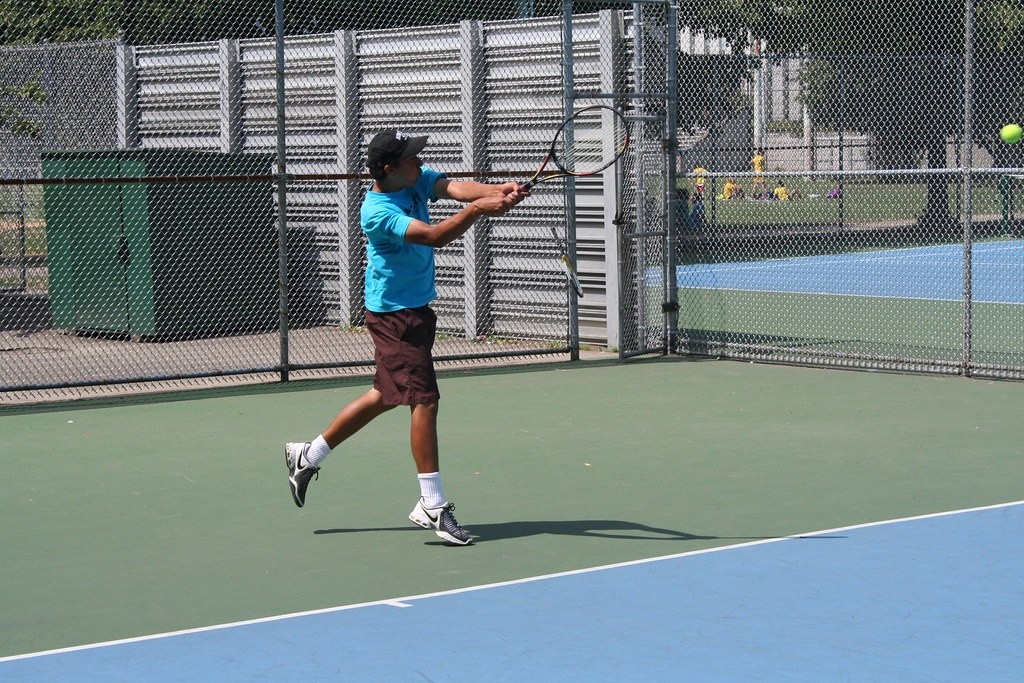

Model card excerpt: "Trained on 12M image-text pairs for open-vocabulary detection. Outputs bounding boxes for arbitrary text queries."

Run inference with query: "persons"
[285,131,531,543]
[690,148,843,202]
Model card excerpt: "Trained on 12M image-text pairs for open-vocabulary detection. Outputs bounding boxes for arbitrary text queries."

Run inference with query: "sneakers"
[285,442,321,508]
[409,497,473,545]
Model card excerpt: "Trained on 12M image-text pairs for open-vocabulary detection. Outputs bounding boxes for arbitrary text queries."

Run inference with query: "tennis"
[1000,123,1023,145]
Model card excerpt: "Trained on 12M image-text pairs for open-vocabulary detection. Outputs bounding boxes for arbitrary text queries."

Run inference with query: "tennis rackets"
[551,227,584,299]
[520,103,631,195]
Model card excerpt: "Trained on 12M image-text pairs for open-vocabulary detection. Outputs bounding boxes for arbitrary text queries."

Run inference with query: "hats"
[366,131,429,171]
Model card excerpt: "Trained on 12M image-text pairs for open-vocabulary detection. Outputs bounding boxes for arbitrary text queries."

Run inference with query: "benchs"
[644,122,717,175]
